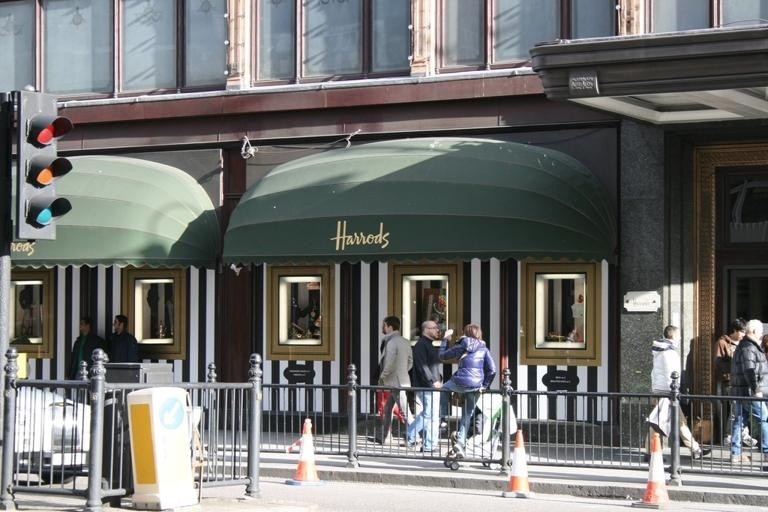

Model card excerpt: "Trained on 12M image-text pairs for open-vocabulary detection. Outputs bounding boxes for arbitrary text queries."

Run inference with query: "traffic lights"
[18,89,73,242]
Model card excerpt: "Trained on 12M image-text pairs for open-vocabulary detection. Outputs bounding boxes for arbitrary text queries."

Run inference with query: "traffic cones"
[286,418,325,486]
[501,429,535,499]
[630,432,680,509]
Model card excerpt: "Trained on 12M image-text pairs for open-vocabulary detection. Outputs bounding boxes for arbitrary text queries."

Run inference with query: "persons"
[364,316,517,458]
[712,317,768,463]
[644,325,712,462]
[104,315,139,362]
[70,318,105,380]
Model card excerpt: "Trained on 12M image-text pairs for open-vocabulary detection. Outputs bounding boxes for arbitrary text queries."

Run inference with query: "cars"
[15,386,124,485]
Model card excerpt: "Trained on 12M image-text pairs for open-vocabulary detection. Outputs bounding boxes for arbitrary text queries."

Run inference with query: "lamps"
[240,136,258,160]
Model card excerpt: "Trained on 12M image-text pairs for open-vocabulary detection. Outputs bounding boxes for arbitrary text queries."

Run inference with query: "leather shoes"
[693,450,710,459]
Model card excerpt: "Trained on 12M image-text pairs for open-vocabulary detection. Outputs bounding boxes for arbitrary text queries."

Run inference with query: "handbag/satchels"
[451,393,465,406]
[649,397,671,437]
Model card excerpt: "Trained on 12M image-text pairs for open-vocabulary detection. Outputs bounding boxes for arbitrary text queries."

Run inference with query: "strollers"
[444,388,516,471]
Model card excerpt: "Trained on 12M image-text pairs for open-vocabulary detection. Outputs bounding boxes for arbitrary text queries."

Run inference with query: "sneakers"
[732,454,751,462]
[453,444,465,458]
[368,437,438,452]
